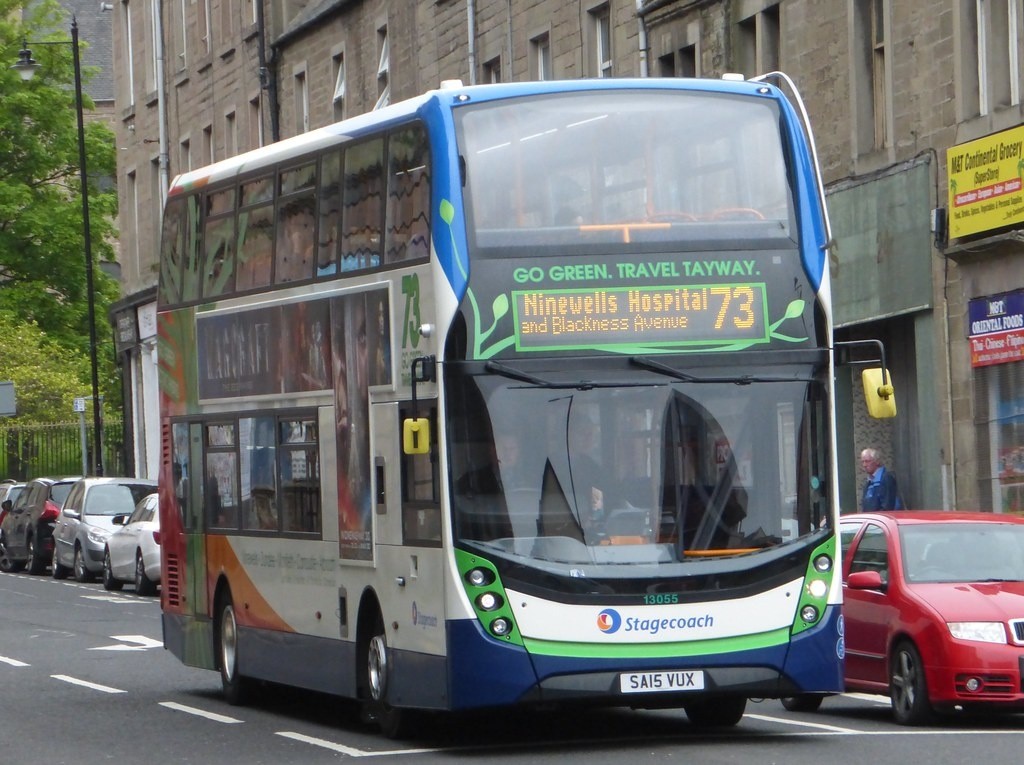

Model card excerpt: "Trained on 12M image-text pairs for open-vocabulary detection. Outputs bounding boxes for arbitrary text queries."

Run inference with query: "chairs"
[920,539,975,582]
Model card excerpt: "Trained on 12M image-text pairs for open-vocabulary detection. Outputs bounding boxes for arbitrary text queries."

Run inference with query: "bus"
[155,69,898,741]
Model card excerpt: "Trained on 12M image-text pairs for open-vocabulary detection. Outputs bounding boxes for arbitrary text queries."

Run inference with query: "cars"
[50,478,158,581]
[0,475,91,575]
[780,508,1023,725]
[103,493,162,596]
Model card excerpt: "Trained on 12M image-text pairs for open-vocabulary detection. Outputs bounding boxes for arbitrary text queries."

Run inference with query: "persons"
[861,448,904,511]
[455,430,535,539]
[543,413,605,516]
